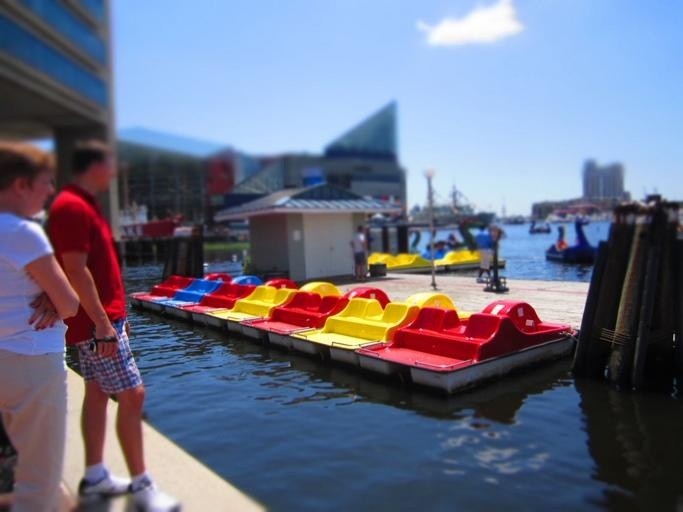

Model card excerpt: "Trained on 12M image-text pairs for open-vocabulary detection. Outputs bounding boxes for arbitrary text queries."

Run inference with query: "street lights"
[425,166,436,286]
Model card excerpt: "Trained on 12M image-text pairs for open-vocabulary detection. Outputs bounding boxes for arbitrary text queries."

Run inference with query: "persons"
[350,225,368,282]
[0,141,82,512]
[49,137,181,512]
[475,223,493,283]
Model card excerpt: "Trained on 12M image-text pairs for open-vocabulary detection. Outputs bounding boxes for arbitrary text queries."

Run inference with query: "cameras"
[90,336,98,354]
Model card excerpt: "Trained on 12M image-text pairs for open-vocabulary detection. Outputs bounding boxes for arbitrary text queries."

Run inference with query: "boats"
[289,292,476,369]
[127,272,343,334]
[545,215,604,262]
[367,233,507,273]
[354,301,578,396]
[237,287,392,350]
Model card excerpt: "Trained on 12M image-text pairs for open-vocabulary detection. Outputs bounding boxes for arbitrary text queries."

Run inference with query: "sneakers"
[79,475,131,496]
[132,476,180,512]
